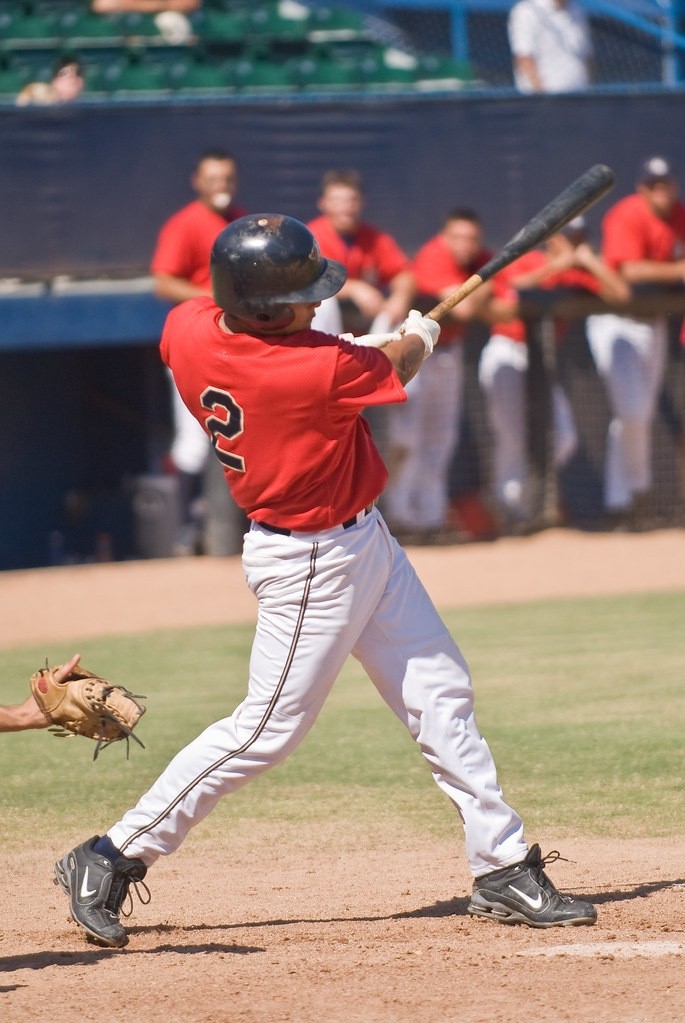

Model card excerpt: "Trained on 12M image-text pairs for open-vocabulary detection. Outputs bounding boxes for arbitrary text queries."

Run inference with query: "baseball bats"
[422,162,617,320]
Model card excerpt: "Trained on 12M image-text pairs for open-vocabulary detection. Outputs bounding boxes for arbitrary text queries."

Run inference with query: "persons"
[12,58,85,107]
[0,654,143,742]
[148,152,685,558]
[506,0,599,95]
[53,213,597,948]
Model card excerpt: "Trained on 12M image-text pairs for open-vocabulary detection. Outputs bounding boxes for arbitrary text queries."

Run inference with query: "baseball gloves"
[30,663,146,741]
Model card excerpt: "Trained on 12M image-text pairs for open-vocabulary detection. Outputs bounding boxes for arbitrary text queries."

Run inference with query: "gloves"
[400,309,442,358]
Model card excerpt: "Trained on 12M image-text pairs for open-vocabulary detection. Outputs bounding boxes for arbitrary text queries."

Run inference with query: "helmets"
[210,214,348,329]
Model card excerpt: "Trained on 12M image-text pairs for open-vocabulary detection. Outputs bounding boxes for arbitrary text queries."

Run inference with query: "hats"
[635,159,674,189]
[560,214,587,242]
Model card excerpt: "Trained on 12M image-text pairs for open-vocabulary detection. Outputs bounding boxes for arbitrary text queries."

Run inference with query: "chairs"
[0,0,480,93]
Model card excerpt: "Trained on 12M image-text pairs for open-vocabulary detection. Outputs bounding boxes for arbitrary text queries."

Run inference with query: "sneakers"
[466,843,598,926]
[52,835,147,947]
